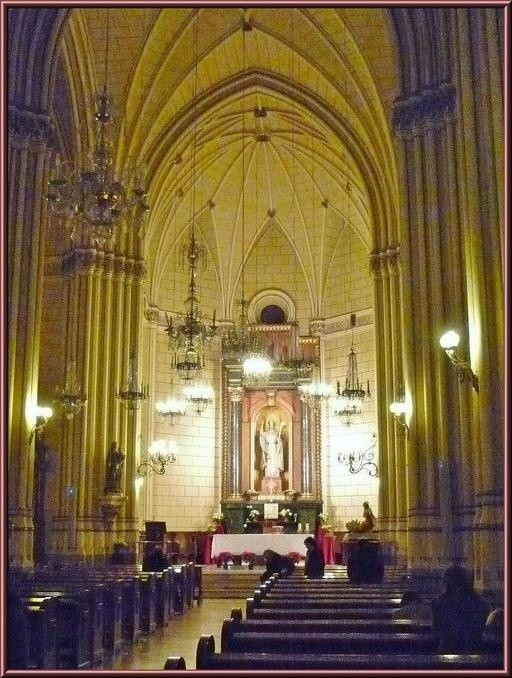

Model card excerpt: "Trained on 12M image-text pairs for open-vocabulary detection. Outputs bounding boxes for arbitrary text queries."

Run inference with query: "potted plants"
[248,509,261,522]
[319,513,333,525]
[243,551,256,570]
[212,512,226,532]
[217,552,233,570]
[206,524,216,535]
[321,524,332,536]
[285,552,302,566]
[279,509,292,522]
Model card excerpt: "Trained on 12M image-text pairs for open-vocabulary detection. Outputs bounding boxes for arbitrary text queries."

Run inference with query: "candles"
[298,523,302,530]
[305,523,309,529]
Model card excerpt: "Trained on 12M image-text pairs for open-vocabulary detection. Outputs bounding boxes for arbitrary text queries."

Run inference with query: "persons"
[304,537,325,579]
[432,565,486,656]
[487,608,503,625]
[392,591,431,620]
[263,548,286,576]
[259,420,286,477]
[347,539,384,585]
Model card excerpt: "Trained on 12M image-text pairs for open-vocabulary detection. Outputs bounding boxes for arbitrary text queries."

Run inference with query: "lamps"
[29,407,52,444]
[183,384,214,416]
[440,329,480,394]
[43,91,151,231]
[114,360,150,416]
[336,432,379,477]
[167,353,207,386]
[336,351,372,401]
[50,360,89,423]
[137,439,179,478]
[335,405,363,426]
[163,229,221,351]
[241,352,272,389]
[220,298,263,362]
[389,401,409,440]
[154,396,187,427]
[298,384,334,403]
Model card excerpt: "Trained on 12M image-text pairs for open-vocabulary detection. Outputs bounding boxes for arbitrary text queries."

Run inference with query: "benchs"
[220,617,501,654]
[40,572,159,636]
[246,597,400,618]
[33,574,142,644]
[171,562,203,616]
[24,581,123,655]
[270,576,414,588]
[60,567,169,627]
[264,581,415,594]
[259,585,436,599]
[230,607,500,631]
[25,583,107,666]
[273,568,407,578]
[9,593,61,669]
[195,634,504,671]
[19,589,92,670]
[253,591,400,607]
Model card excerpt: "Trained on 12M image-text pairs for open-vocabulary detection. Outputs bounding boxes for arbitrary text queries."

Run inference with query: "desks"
[210,534,317,567]
[133,521,173,571]
